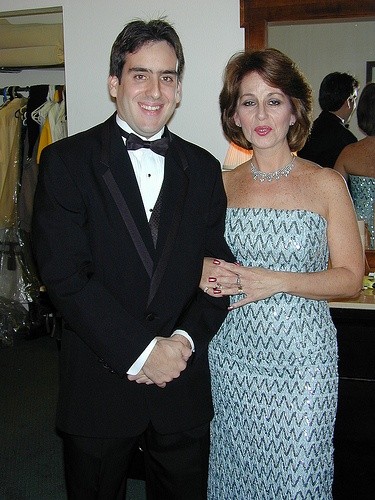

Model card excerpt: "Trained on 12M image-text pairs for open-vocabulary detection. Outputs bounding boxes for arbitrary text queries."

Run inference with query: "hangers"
[1,84,67,126]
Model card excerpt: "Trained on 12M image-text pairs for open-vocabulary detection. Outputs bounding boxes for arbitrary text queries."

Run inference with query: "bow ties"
[122,134,173,152]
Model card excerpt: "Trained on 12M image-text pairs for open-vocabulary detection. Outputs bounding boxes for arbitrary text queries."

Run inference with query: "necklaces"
[250,153,296,183]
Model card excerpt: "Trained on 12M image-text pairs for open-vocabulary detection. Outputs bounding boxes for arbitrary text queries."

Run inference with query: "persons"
[297,71,361,170]
[30,19,237,500]
[199,48,365,500]
[334,83,375,250]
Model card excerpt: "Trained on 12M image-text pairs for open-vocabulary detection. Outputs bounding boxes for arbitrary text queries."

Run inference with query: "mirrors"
[244,0,375,252]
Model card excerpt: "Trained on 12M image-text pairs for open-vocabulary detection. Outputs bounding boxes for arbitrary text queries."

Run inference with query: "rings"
[237,276,241,285]
[238,287,242,295]
[216,283,221,288]
[204,287,209,292]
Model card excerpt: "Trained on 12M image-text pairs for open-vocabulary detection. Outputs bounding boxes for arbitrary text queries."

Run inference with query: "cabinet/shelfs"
[326,288,375,383]
